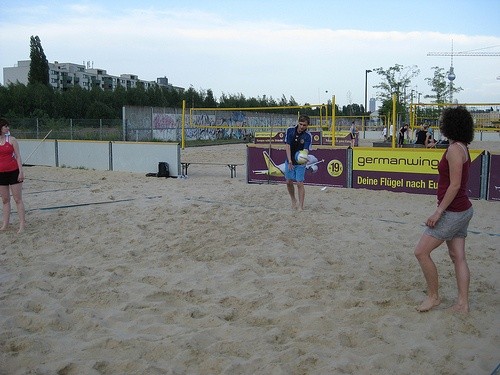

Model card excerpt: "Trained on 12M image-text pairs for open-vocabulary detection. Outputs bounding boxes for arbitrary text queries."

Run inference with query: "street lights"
[403,85,408,113]
[397,82,402,129]
[364,70,373,131]
[417,93,423,116]
[410,89,415,103]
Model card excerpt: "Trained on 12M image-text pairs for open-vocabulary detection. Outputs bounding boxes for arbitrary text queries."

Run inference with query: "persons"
[383,122,412,148]
[414,107,475,312]
[350,121,355,146]
[0,119,25,234]
[415,125,449,148]
[285,115,312,210]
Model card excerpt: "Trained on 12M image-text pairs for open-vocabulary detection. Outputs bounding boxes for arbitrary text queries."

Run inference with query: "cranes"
[427,44,500,57]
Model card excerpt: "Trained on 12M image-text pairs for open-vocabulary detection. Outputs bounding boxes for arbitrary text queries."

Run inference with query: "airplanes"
[253,150,326,179]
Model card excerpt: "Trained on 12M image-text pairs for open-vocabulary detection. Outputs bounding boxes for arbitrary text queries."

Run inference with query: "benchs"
[179,146,246,178]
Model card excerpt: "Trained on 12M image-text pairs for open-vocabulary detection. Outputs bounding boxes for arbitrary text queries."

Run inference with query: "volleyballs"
[295,150,308,164]
